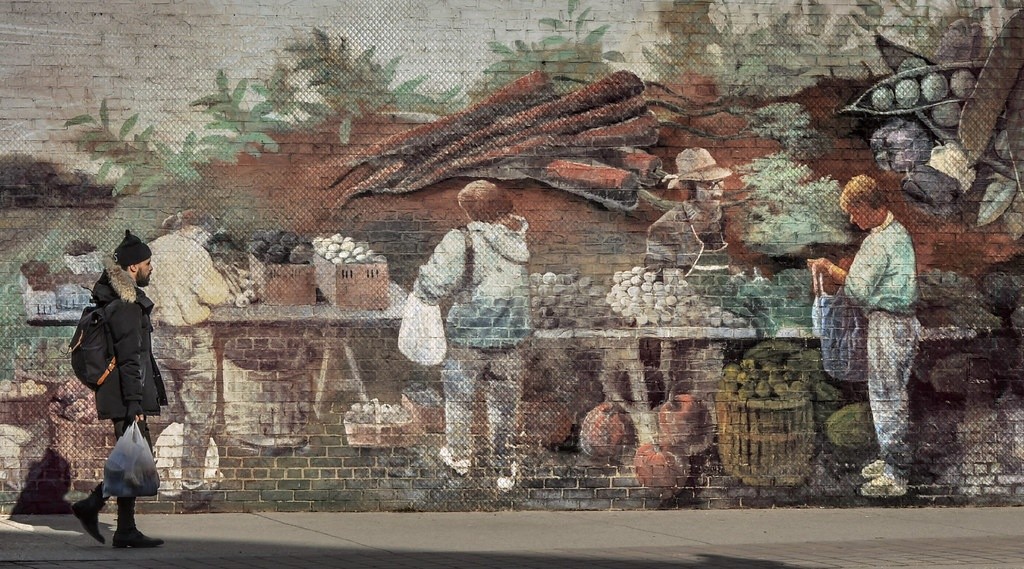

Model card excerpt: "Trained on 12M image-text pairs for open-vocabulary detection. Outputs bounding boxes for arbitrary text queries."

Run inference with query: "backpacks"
[67,299,128,394]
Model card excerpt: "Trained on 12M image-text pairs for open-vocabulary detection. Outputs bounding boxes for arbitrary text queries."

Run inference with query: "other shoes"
[72,489,106,545]
[112,529,165,548]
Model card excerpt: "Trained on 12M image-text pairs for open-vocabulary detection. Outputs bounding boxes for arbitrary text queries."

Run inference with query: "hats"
[112,229,152,267]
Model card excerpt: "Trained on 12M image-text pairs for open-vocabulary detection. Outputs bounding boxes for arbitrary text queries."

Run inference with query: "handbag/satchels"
[102,422,160,497]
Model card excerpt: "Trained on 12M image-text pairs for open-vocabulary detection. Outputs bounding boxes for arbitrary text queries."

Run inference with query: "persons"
[70,229,169,549]
